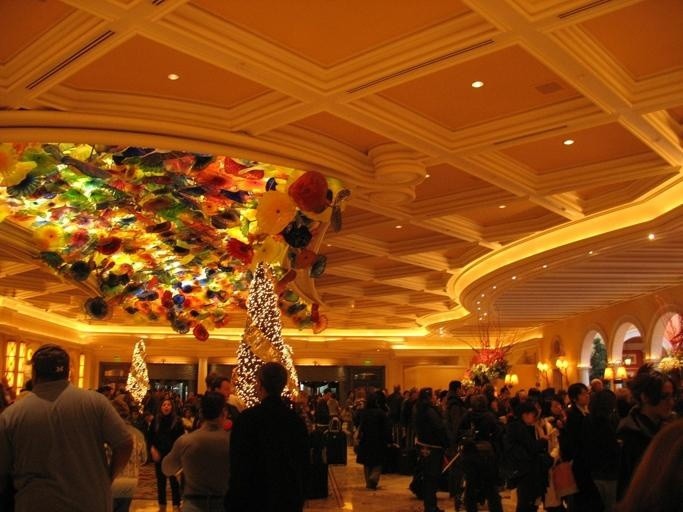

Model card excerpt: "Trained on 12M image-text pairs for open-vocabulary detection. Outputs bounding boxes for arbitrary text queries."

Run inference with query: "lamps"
[503,356,627,391]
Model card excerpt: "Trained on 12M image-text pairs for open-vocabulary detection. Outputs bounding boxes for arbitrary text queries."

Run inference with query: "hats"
[27,343,70,374]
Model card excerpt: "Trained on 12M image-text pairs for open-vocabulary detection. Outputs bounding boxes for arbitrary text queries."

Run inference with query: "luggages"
[327,419,347,464]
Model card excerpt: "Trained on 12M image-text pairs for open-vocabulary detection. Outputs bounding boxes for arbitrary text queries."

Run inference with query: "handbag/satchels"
[545,471,562,509]
[553,463,576,496]
[112,478,139,499]
